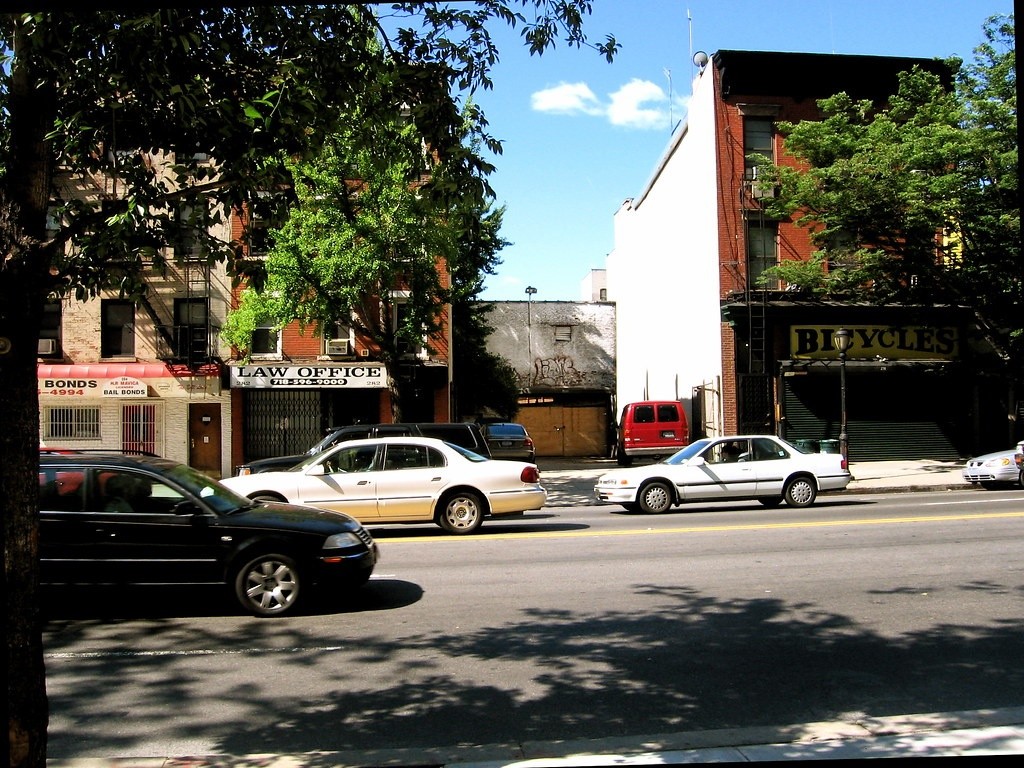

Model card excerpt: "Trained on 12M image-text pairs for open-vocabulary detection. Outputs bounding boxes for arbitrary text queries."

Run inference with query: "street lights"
[834,327,853,469]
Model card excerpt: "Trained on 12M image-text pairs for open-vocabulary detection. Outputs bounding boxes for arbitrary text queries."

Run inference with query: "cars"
[481,423,536,464]
[595,434,852,514]
[215,436,549,536]
[962,448,1022,490]
[39,447,161,498]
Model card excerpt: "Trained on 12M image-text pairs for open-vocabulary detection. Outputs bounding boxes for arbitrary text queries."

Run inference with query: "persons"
[709,446,737,463]
[326,448,374,475]
[107,473,138,515]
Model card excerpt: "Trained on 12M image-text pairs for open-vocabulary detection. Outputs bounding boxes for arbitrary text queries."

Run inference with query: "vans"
[615,400,690,465]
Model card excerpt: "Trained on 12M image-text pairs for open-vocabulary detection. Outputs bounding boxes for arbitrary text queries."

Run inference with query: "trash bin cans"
[816,439,840,454]
[796,438,819,452]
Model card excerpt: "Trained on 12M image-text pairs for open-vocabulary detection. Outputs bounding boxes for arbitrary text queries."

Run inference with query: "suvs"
[235,421,492,475]
[39,447,380,617]
